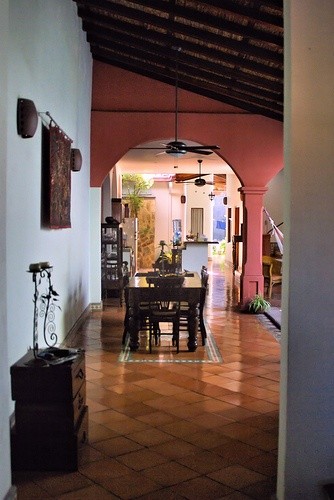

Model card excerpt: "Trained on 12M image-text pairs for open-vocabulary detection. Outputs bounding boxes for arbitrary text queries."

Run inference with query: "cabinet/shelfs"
[10,348,88,472]
[263,234,271,255]
[101,223,123,308]
[113,204,131,223]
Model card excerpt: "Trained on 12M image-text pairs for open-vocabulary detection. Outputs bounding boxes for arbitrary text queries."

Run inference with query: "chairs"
[123,266,209,354]
[262,255,282,299]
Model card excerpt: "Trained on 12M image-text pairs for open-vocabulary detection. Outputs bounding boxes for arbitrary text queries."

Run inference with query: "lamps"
[209,192,215,201]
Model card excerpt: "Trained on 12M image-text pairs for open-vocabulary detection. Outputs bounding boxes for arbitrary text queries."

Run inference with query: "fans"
[130,49,221,157]
[175,160,213,187]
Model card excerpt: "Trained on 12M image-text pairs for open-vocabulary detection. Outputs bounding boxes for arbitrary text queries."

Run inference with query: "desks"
[124,269,203,352]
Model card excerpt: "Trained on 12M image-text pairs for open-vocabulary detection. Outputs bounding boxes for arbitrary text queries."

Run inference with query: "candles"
[29,264,40,270]
[41,262,49,267]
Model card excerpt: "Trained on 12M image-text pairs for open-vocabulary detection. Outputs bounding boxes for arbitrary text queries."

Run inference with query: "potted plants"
[248,294,271,314]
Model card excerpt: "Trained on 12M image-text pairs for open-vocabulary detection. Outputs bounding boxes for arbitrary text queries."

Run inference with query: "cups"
[204,238,208,241]
